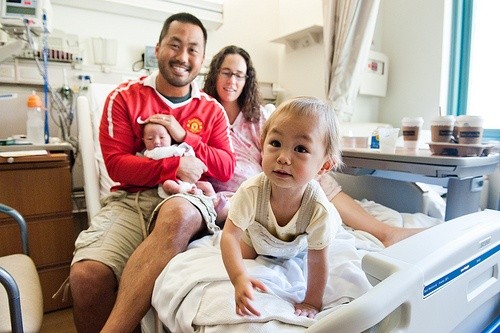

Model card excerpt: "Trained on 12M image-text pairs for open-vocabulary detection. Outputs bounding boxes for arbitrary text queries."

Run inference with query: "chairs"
[0,202,43,333]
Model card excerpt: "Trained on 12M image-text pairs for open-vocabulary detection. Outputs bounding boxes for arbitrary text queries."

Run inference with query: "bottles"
[26,90,45,144]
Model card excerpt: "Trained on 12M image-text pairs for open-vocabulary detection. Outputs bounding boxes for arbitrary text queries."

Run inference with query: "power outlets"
[62,85,69,95]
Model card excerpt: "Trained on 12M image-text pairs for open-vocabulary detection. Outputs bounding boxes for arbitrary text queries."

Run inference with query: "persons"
[69,12,236,333]
[142,123,227,213]
[204,45,436,248]
[219,96,347,318]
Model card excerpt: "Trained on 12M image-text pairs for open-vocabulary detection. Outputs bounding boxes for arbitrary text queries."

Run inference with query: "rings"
[162,117,166,121]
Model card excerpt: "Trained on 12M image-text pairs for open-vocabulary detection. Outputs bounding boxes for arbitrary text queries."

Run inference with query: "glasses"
[219,68,249,81]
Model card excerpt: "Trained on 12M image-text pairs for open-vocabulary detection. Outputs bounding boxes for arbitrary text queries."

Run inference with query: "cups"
[429,114,456,143]
[401,117,423,149]
[378,127,400,154]
[457,115,484,145]
[452,116,460,137]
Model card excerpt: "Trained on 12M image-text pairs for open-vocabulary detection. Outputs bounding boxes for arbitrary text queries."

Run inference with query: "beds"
[76,82,500,333]
